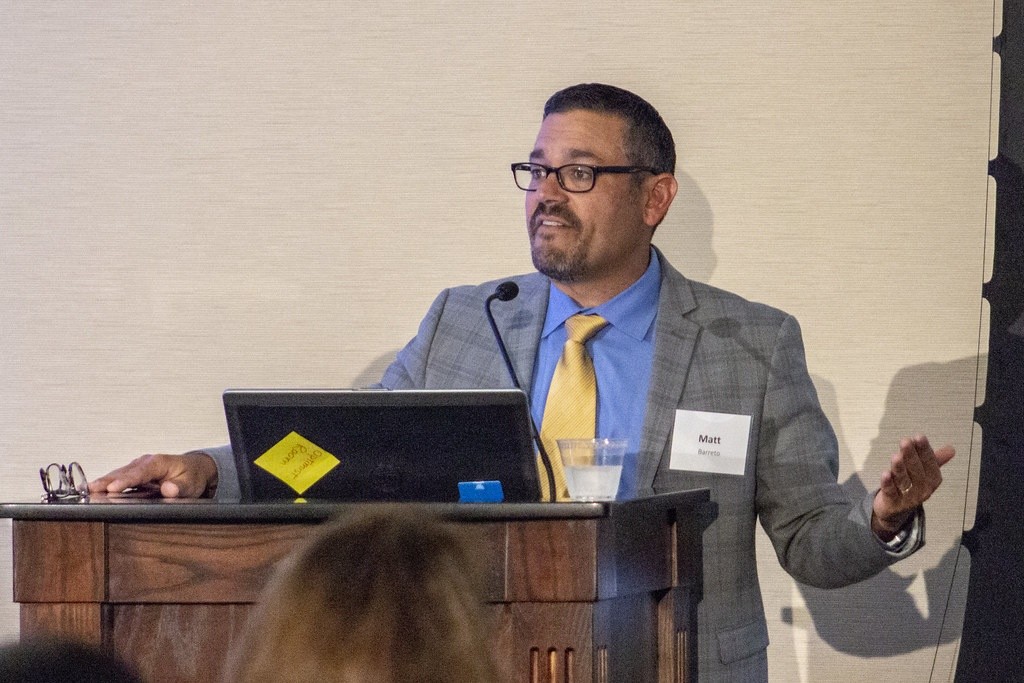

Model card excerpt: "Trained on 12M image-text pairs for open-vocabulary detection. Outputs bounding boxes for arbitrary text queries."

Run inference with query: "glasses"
[40,462,90,503]
[512,162,663,193]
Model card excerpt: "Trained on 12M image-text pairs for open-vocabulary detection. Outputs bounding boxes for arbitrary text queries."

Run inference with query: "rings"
[898,483,912,494]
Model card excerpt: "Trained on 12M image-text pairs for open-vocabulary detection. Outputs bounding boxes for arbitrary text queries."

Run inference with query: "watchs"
[887,512,914,546]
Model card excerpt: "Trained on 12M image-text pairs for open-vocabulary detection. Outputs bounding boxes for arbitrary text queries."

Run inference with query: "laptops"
[221,389,543,504]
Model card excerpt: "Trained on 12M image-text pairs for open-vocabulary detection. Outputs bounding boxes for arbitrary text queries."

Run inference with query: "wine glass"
[556,437,628,504]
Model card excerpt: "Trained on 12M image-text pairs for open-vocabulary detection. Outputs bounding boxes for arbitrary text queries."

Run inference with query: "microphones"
[485,282,556,505]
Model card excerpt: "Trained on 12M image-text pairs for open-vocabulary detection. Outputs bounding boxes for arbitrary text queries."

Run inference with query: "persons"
[84,82,955,683]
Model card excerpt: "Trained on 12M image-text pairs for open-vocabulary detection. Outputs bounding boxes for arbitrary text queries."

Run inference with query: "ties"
[534,312,613,502]
